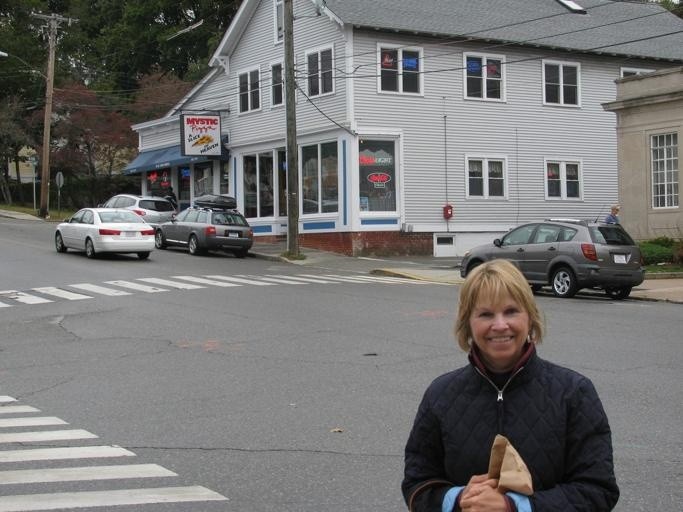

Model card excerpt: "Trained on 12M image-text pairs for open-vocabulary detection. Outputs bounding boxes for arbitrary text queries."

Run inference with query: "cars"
[460,217,646,301]
[55,207,156,260]
[99,194,177,239]
[155,196,256,257]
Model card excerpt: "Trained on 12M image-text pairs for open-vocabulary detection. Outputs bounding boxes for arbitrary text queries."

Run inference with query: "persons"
[161,187,177,209]
[401,259,620,512]
[606,205,620,223]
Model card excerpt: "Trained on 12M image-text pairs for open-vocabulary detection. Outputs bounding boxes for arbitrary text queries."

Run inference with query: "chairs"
[543,234,558,242]
